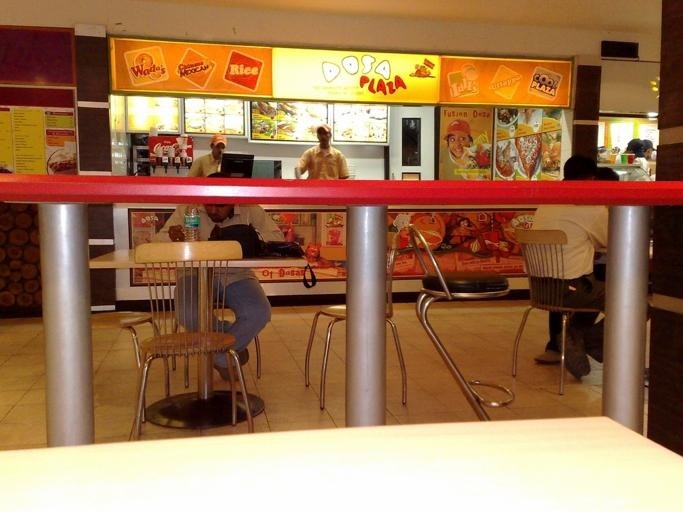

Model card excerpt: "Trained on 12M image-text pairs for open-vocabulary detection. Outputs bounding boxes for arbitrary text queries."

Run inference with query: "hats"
[628,139,657,157]
[317,125,331,132]
[442,121,470,140]
[210,135,227,147]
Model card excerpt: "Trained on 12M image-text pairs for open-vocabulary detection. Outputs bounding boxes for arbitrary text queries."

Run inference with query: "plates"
[409,214,446,239]
[495,108,560,180]
[46,147,77,175]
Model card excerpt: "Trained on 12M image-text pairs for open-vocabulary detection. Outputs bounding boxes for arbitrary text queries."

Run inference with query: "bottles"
[182,203,202,242]
[283,227,294,243]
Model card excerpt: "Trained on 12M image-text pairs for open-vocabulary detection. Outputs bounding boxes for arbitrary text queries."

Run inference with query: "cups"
[609,153,635,165]
[167,147,175,157]
[324,229,340,246]
[154,146,163,156]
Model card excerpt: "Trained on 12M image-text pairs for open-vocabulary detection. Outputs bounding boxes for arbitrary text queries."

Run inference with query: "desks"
[89,249,308,430]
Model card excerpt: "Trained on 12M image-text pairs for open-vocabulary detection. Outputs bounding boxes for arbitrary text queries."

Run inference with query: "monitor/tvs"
[220,153,255,177]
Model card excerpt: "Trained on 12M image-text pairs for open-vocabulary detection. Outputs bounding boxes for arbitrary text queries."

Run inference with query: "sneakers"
[238,348,249,365]
[215,366,239,382]
[534,321,604,378]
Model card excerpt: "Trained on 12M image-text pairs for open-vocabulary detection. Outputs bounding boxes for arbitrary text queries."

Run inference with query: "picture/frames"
[402,172,421,180]
[401,117,421,166]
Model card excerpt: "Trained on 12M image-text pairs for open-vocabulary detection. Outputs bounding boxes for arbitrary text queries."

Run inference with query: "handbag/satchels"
[209,224,261,257]
[262,240,303,257]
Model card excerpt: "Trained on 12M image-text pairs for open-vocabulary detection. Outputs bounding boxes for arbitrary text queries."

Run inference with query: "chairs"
[171,307,261,388]
[304,231,407,410]
[512,227,604,396]
[91,311,170,422]
[134,241,254,442]
[407,223,515,422]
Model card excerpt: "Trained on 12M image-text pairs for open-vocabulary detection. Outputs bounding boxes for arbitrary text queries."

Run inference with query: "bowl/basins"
[412,226,442,252]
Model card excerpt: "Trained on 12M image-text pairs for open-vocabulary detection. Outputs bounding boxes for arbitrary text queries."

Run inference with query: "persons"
[534,166,620,363]
[153,170,285,383]
[295,123,351,179]
[183,134,227,178]
[523,155,609,380]
[442,120,473,162]
[614,139,644,163]
[638,139,656,174]
